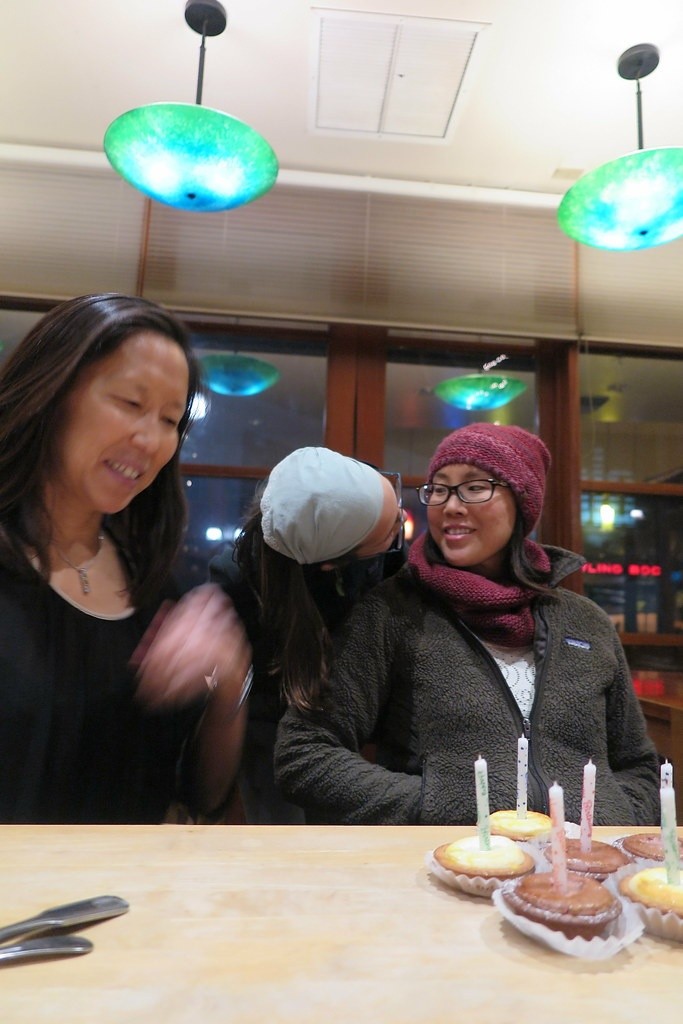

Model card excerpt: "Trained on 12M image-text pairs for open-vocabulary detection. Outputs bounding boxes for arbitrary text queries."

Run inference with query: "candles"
[475,754,493,850]
[580,754,596,854]
[660,758,672,789]
[516,732,528,820]
[660,787,681,884]
[549,781,567,897]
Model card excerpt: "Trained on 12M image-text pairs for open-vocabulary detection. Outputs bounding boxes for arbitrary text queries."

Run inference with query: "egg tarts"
[544,839,637,880]
[434,836,535,879]
[490,810,551,840]
[613,833,683,864]
[502,871,622,940]
[618,866,683,918]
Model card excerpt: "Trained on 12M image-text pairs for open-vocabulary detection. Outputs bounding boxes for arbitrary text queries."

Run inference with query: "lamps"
[196,351,280,397]
[434,369,527,412]
[556,43,683,253]
[103,0,280,214]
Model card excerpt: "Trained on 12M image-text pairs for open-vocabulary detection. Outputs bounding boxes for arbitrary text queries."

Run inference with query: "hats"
[426,422,551,537]
[261,447,384,565]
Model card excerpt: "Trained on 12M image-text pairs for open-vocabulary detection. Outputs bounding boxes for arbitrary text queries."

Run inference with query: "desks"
[0,822,683,1024]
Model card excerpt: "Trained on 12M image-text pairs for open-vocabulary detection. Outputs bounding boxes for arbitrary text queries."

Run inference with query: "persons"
[0,290,255,825]
[222,445,409,826]
[272,421,661,826]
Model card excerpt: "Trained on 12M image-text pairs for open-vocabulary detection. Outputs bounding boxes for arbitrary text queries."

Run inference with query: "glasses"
[360,472,403,557]
[416,479,511,506]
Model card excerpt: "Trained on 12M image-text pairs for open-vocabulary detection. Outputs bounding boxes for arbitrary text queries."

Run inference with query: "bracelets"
[226,663,256,721]
